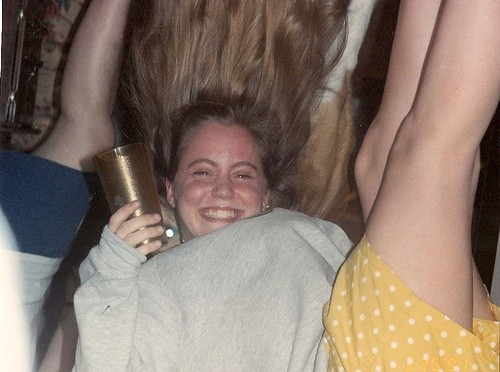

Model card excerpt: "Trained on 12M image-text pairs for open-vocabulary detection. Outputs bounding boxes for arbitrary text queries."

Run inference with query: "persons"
[68,1,500,372]
[1,0,131,372]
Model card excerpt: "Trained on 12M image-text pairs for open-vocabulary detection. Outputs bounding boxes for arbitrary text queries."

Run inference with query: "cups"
[93,140,168,256]
